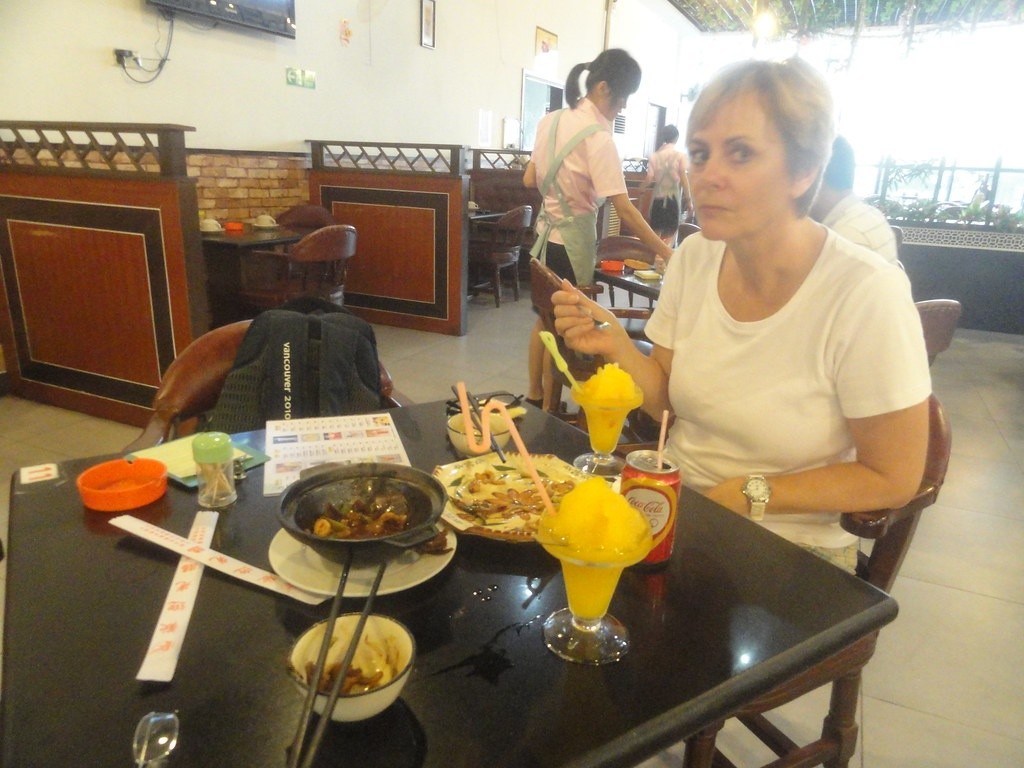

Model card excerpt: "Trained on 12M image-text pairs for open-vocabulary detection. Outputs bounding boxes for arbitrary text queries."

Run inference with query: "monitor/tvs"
[143,0,296,38]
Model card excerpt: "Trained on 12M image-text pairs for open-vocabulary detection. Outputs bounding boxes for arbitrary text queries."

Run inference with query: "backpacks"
[196,295,381,435]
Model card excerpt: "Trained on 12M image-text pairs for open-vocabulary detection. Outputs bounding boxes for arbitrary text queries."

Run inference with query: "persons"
[641,124,692,236]
[523,48,674,424]
[552,58,932,573]
[806,134,898,263]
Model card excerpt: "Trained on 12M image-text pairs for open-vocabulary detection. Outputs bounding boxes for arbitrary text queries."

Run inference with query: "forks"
[534,259,613,333]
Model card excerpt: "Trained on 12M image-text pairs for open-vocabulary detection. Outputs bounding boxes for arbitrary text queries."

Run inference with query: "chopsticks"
[451,385,507,463]
[288,550,388,768]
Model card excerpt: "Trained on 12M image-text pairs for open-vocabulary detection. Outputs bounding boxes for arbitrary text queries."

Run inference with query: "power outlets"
[111,49,143,68]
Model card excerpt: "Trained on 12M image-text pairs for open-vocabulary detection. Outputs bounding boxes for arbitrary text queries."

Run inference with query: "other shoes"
[526,397,567,412]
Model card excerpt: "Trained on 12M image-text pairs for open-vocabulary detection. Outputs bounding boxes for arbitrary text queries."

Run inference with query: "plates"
[268,520,458,598]
[251,222,280,229]
[199,228,226,234]
[432,450,596,543]
[468,206,481,210]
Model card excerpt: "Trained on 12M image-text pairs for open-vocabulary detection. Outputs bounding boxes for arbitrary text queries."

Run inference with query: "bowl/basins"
[447,410,512,456]
[276,461,449,561]
[284,611,417,720]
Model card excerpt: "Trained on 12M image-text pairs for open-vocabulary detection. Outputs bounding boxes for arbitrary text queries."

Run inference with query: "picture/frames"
[421,0,436,50]
[534,25,558,58]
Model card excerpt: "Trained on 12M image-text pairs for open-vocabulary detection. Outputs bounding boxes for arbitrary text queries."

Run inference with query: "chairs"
[277,204,335,254]
[614,393,947,768]
[125,319,415,455]
[465,205,965,442]
[238,226,362,317]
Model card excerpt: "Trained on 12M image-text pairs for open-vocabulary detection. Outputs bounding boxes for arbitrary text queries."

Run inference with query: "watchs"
[742,473,770,519]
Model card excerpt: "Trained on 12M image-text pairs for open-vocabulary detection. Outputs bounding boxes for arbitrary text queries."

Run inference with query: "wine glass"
[534,502,653,667]
[569,377,644,478]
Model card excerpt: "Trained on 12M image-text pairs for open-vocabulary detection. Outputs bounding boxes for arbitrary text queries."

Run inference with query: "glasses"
[446,394,524,415]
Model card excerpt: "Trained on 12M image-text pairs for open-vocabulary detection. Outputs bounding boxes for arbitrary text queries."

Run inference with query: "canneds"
[620,449,681,567]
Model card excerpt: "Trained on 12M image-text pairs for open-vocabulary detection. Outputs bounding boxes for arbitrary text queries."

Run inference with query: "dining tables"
[594,265,666,300]
[467,208,509,305]
[198,221,315,327]
[0,390,898,768]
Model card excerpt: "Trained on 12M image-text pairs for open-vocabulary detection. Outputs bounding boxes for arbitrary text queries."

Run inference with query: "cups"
[468,200,478,207]
[256,214,276,225]
[201,219,221,230]
